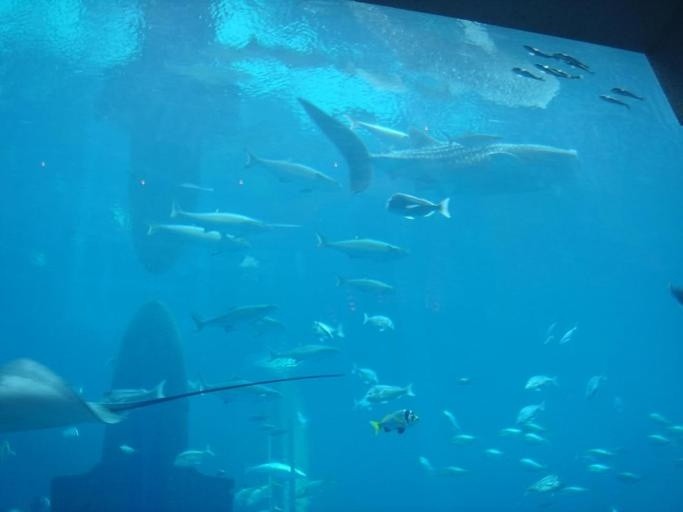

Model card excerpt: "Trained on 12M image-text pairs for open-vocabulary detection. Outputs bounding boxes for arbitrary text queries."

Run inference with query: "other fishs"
[244,96,577,193]
[0,281,683,512]
